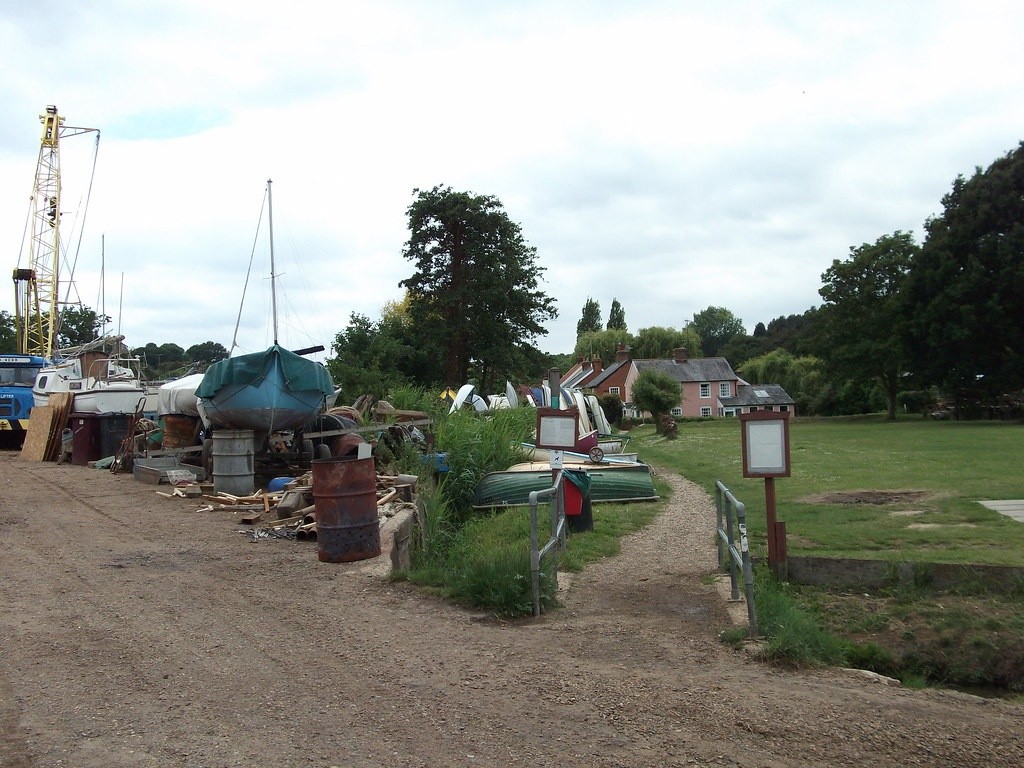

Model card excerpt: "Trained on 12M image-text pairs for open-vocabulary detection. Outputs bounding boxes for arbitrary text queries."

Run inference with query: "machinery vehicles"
[1,107,100,449]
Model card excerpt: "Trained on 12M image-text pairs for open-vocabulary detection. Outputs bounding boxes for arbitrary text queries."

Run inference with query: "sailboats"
[32,176,342,438]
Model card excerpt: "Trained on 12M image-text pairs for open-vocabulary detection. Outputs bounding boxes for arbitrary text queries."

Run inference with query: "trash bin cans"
[95,412,129,459]
[67,413,101,465]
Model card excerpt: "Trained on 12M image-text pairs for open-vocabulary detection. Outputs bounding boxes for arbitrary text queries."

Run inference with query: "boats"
[441,379,661,508]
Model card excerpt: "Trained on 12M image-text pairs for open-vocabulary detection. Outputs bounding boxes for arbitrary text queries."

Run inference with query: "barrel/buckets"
[162,415,195,449]
[311,455,381,563]
[212,428,255,496]
[554,468,594,533]
